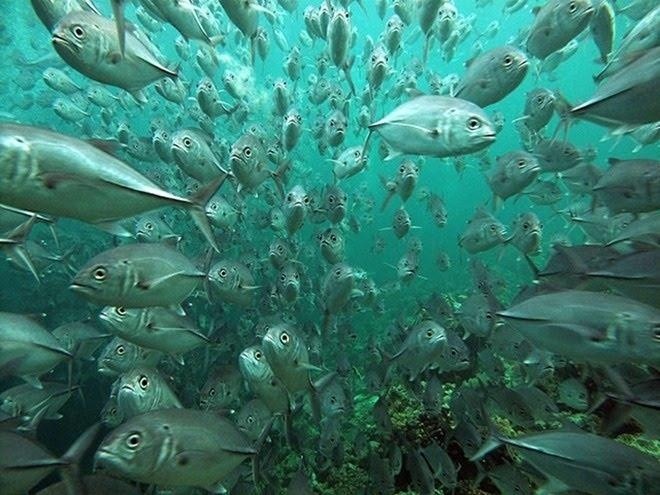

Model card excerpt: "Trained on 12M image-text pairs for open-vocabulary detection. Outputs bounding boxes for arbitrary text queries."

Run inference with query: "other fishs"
[0,0,660,495]
